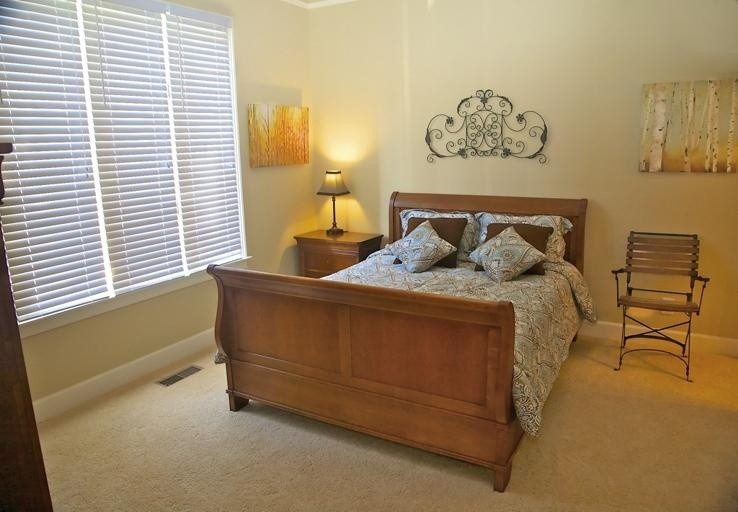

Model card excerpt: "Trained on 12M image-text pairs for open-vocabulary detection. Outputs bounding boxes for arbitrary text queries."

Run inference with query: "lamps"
[316,170,350,235]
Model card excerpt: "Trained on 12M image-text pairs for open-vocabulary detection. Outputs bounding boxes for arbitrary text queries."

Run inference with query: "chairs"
[612,231,710,382]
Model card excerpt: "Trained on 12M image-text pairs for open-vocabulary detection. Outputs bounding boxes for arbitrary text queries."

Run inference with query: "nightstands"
[294,230,384,279]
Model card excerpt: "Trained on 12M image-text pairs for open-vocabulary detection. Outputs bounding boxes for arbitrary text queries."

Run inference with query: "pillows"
[388,208,574,286]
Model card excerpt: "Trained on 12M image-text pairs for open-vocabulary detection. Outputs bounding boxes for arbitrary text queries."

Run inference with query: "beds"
[207,191,589,493]
